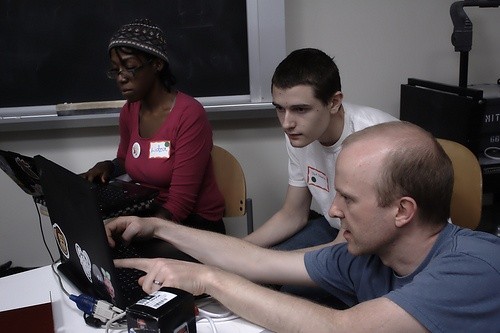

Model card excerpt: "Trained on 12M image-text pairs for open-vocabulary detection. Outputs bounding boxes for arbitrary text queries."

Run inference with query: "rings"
[152,280,163,285]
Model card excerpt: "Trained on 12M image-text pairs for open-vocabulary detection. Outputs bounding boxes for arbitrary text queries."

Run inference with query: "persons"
[79,18,227,263]
[102,121,500,333]
[241,48,452,252]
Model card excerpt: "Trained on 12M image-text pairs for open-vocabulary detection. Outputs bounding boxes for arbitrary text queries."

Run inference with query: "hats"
[107,16,172,64]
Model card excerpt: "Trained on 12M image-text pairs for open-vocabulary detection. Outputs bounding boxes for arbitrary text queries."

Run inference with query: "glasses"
[104,61,149,80]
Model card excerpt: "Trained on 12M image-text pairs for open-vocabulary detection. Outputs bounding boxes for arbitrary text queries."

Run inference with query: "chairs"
[210,146,254,234]
[435,138,483,231]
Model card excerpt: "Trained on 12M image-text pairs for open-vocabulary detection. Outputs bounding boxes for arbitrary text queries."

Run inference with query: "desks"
[0,260,265,333]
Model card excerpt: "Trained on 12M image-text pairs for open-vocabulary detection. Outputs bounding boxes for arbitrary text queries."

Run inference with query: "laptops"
[33,154,210,315]
[0,149,159,217]
